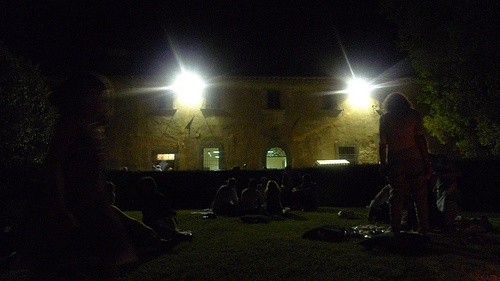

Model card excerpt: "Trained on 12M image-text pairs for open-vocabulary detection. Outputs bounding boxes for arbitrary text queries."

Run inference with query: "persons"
[380,94,434,237]
[427,166,470,229]
[5,70,180,280]
[214,167,322,216]
